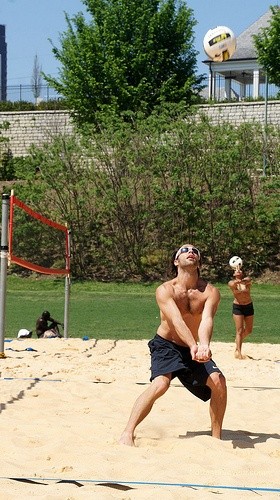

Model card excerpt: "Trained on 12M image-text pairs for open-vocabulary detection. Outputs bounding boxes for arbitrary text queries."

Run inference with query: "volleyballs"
[202,25,239,63]
[229,255,243,271]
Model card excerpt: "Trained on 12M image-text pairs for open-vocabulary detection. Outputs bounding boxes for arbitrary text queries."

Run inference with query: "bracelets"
[240,278,246,282]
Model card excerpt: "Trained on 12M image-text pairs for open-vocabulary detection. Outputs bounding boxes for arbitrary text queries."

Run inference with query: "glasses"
[176,248,200,259]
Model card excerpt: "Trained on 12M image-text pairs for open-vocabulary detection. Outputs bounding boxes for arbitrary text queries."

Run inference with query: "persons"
[36,311,64,338]
[228,269,255,360]
[116,243,227,449]
